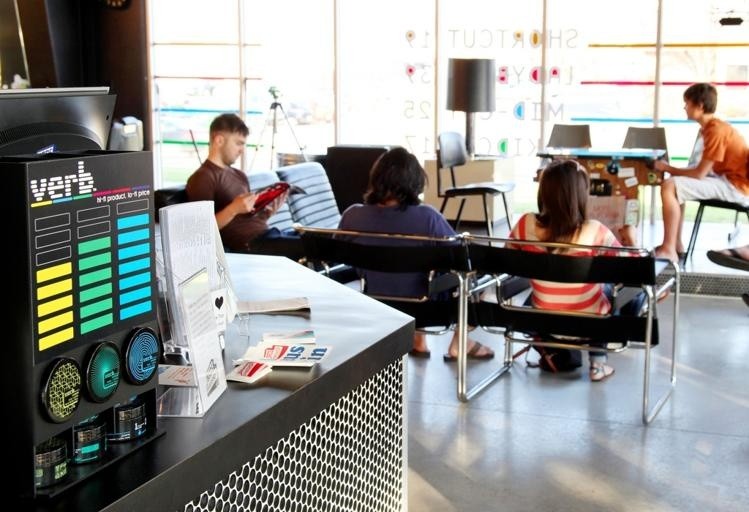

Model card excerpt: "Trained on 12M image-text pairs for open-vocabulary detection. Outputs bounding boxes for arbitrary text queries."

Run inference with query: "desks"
[538,149,667,226]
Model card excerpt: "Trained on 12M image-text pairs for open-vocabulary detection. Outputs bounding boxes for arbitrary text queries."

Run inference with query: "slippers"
[650,244,689,262]
[443,342,495,362]
[409,347,431,359]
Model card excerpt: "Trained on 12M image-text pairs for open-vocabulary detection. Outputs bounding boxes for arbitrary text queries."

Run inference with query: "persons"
[653,82,749,263]
[502,158,638,381]
[182,114,316,268]
[334,146,495,363]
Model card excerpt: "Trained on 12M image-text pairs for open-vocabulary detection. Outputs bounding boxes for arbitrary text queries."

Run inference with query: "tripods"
[247,100,309,171]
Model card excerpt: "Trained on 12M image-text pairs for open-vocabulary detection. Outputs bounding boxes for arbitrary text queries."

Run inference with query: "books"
[155,201,223,345]
[244,183,308,217]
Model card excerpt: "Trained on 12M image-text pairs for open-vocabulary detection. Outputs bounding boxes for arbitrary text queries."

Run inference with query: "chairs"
[682,198,749,266]
[547,124,592,148]
[434,130,513,247]
[621,126,669,161]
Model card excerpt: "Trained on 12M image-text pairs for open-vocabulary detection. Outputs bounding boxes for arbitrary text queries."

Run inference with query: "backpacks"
[524,330,583,372]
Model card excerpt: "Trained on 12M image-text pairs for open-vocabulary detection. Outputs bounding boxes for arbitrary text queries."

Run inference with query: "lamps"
[445,57,496,158]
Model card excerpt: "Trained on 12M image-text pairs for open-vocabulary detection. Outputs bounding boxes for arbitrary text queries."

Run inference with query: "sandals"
[588,364,616,383]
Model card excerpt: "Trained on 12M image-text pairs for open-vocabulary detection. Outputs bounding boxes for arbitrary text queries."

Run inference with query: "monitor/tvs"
[1,86,117,154]
